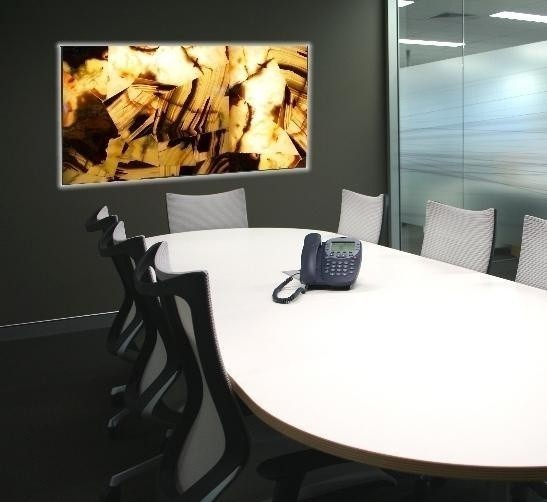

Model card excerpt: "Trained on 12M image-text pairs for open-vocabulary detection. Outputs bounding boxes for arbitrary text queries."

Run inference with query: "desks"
[126,215,547,477]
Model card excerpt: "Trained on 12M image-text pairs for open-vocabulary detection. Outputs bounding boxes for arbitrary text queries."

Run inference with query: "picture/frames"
[52,42,312,190]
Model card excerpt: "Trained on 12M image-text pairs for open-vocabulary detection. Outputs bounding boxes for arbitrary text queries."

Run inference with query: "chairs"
[129,242,303,498]
[108,226,165,388]
[327,188,387,244]
[513,212,547,294]
[163,189,256,231]
[418,199,497,272]
[93,207,139,340]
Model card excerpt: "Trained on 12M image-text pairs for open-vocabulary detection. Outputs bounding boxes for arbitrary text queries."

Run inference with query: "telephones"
[300,233,362,290]
